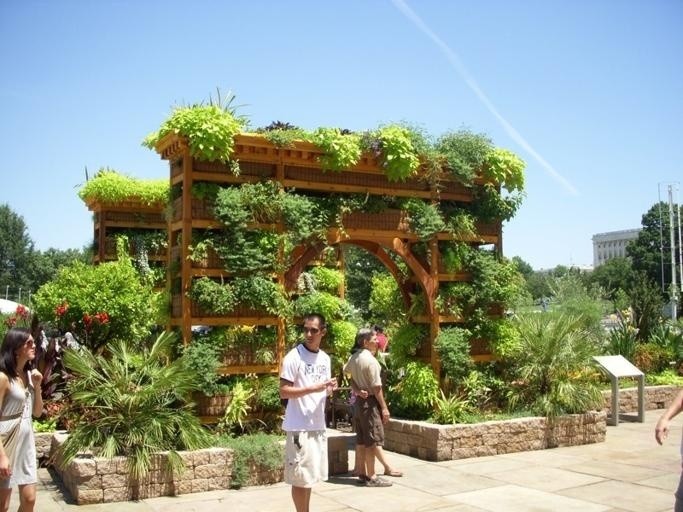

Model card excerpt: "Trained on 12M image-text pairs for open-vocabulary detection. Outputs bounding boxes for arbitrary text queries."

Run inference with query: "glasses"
[25,340,34,347]
[302,327,321,333]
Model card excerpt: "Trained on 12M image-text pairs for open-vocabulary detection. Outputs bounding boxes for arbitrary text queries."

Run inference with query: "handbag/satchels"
[0,423,20,481]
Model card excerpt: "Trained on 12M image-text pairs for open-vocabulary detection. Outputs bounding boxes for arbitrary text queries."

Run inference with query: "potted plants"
[153,104,528,415]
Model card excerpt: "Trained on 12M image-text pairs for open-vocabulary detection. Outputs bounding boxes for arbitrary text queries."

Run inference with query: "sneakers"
[359,469,403,487]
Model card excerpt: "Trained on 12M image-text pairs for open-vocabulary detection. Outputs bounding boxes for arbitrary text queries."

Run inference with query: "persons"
[0,326,45,512]
[278,312,338,512]
[344,327,379,487]
[349,332,405,477]
[374,324,389,353]
[655,387,683,512]
